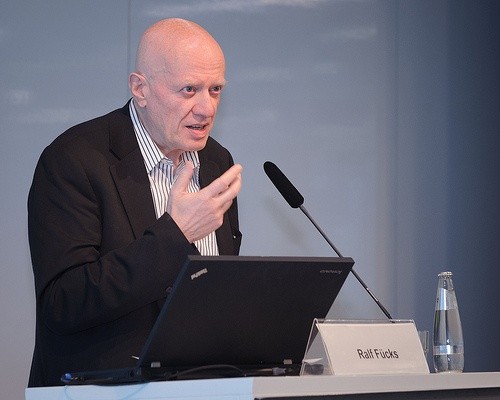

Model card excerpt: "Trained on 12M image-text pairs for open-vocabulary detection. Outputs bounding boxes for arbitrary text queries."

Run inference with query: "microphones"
[262,161,395,324]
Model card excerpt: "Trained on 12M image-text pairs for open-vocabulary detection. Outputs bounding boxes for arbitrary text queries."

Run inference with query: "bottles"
[432,272,464,373]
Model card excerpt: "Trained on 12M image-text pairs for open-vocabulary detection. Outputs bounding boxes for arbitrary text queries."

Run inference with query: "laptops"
[63,254,355,384]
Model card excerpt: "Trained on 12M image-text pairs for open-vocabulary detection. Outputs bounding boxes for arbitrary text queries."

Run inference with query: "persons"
[27,18,242,387]
